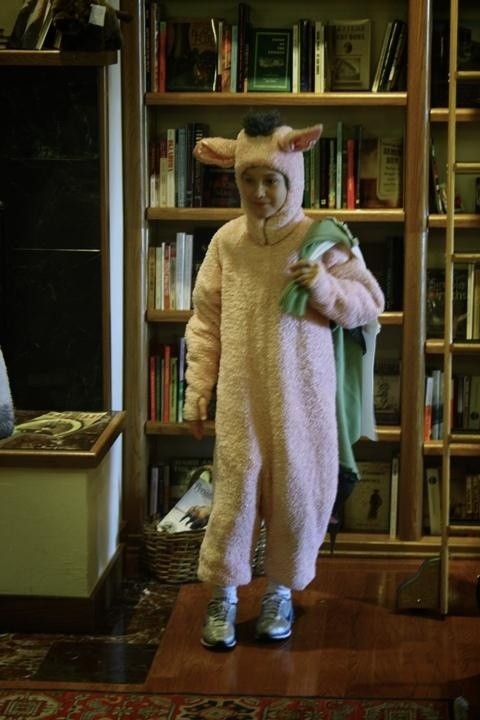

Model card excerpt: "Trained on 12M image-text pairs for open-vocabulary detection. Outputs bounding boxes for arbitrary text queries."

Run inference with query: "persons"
[181,108,387,652]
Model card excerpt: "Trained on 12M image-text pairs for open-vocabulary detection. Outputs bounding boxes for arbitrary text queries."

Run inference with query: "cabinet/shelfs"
[120,0,480,558]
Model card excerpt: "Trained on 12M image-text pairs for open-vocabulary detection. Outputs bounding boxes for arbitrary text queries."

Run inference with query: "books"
[427,252,480,341]
[421,454,478,536]
[148,123,242,209]
[371,346,400,419]
[362,249,404,310]
[147,228,214,311]
[148,342,216,422]
[424,365,479,438]
[145,457,219,532]
[343,459,389,536]
[142,6,406,91]
[429,138,477,212]
[302,124,404,209]
[7,1,63,51]
[431,17,478,109]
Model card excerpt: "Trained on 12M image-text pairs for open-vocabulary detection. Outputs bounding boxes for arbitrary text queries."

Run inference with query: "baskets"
[142,464,281,584]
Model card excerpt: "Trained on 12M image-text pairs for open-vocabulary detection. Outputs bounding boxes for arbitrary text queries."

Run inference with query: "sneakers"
[200,599,237,649]
[254,592,294,642]
